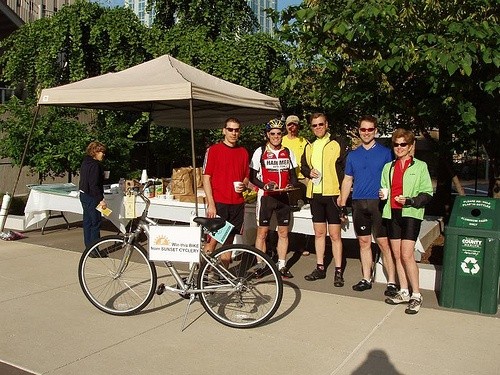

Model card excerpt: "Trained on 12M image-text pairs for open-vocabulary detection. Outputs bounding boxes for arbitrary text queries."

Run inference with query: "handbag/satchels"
[196,166,202,188]
[171,165,194,199]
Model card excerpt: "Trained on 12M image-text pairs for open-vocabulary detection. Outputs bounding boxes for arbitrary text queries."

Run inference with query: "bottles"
[144,177,163,198]
[119,176,125,187]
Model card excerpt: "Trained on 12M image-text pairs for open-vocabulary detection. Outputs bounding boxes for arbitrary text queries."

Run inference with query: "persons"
[436,142,465,220]
[339,115,398,297]
[202,118,250,294]
[249,118,298,278]
[79,142,108,258]
[301,112,348,287]
[281,115,312,255]
[380,127,433,315]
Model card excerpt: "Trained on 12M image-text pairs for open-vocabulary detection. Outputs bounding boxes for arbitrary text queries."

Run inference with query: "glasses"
[393,143,409,147]
[359,128,375,132]
[310,123,326,128]
[226,128,240,133]
[268,132,281,135]
[96,150,105,154]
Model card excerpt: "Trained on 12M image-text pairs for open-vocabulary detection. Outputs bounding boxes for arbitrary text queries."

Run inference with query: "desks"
[22,183,444,283]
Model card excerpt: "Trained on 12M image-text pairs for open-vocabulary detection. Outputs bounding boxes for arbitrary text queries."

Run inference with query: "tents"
[0,53,283,263]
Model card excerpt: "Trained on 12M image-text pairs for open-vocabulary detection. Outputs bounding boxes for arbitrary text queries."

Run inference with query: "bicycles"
[78,180,284,333]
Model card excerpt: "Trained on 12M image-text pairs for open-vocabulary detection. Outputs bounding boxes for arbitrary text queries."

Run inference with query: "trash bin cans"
[441,197,500,315]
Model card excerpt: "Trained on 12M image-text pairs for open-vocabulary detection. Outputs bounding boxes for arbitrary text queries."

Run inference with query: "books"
[95,204,112,217]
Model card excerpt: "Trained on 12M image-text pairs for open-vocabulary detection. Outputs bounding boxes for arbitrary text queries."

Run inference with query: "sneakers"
[304,269,326,281]
[385,290,410,304]
[254,263,271,278]
[352,279,372,291]
[334,271,344,287]
[279,267,293,278]
[405,296,423,314]
[384,283,399,296]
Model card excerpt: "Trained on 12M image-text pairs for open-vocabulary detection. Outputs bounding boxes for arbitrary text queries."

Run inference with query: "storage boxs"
[373,258,436,291]
[4,215,24,230]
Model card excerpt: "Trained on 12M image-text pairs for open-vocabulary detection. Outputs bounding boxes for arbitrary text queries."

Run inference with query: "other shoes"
[218,275,236,285]
[203,278,214,295]
[89,251,107,258]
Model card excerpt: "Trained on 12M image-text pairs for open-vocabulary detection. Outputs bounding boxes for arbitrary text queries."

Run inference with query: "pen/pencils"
[267,179,280,188]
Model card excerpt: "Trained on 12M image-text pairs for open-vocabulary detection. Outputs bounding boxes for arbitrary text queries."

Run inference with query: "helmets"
[266,118,284,132]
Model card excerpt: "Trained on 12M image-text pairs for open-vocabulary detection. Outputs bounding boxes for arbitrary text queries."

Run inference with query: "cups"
[234,182,242,193]
[380,188,388,200]
[141,168,147,184]
[104,171,110,179]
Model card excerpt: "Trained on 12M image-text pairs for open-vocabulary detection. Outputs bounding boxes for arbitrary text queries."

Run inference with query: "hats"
[285,115,300,125]
